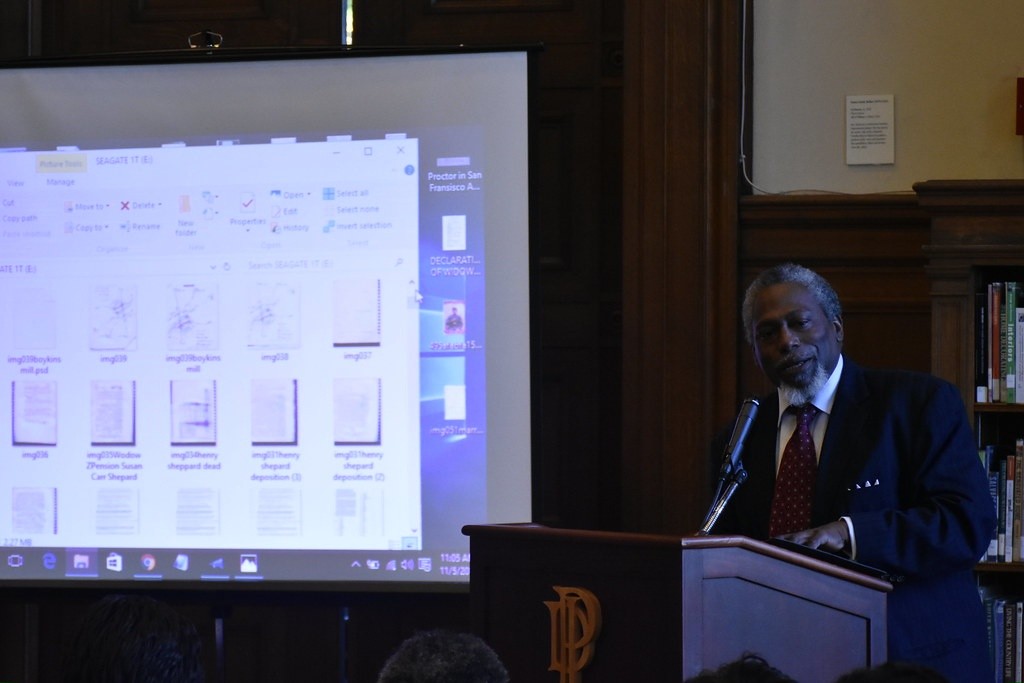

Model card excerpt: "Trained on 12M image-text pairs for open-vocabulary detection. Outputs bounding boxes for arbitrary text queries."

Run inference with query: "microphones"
[718,394,761,482]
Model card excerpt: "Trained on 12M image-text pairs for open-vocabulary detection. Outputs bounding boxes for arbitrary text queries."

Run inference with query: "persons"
[708,261,997,683]
[377,627,510,683]
[58,593,205,683]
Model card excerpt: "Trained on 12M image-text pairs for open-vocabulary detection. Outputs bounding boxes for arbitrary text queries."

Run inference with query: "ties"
[768,403,824,541]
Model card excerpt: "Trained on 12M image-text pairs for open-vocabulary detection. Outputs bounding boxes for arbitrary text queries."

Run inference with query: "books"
[975,281,1024,405]
[976,586,1023,681]
[977,438,1024,564]
